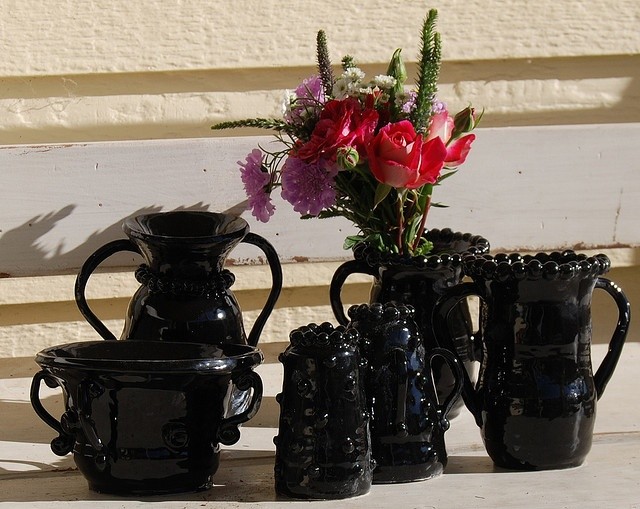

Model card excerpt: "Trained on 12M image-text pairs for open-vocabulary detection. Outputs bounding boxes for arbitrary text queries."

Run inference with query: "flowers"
[211,10,486,257]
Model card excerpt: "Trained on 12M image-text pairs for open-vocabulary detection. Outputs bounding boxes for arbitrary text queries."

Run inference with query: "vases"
[31,341,265,495]
[73,210,283,422]
[274,320,377,502]
[330,228,491,419]
[348,303,443,483]
[437,251,631,469]
